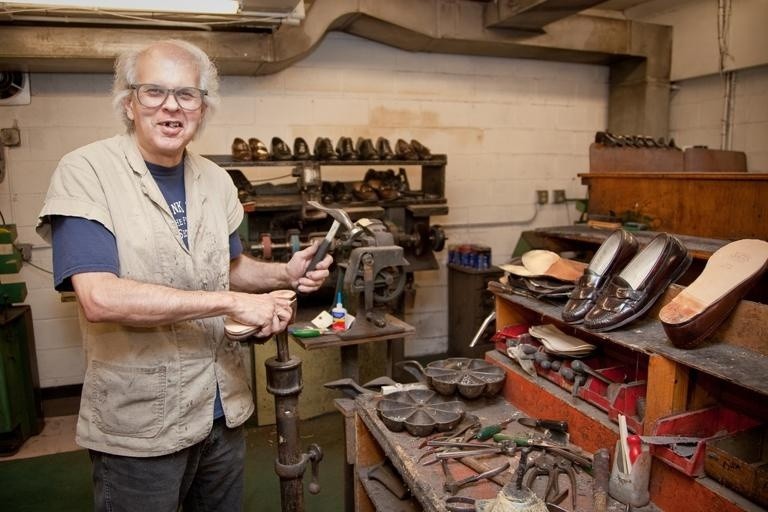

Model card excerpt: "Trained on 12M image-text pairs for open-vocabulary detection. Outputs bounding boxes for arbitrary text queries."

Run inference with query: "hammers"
[295,200,364,297]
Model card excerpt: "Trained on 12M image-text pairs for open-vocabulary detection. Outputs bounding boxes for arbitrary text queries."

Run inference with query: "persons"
[35,40,334,512]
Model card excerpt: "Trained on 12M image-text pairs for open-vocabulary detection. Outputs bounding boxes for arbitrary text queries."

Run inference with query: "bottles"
[448,243,492,271]
[332,288,347,332]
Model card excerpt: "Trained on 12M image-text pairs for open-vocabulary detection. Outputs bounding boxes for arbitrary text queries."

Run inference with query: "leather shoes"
[225,289,297,346]
[377,137,393,160]
[584,233,693,333]
[396,139,419,160]
[411,140,432,159]
[336,137,358,160]
[249,139,268,160]
[356,137,379,159]
[323,178,401,204]
[659,238,768,349]
[232,137,251,161]
[313,137,338,158]
[562,228,639,325]
[295,137,309,158]
[272,137,291,159]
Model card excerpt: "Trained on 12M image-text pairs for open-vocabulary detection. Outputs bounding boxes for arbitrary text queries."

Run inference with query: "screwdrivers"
[476,418,516,441]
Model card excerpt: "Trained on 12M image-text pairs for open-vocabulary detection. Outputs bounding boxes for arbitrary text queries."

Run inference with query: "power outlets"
[536,190,548,204]
[552,189,566,204]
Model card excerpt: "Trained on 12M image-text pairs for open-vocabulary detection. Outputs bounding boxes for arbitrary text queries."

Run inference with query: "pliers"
[417,421,593,512]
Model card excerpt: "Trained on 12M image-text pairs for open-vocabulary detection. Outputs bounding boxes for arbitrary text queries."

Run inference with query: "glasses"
[130,84,209,111]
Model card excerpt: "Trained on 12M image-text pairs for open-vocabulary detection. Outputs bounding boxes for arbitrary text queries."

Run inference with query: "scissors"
[291,328,336,337]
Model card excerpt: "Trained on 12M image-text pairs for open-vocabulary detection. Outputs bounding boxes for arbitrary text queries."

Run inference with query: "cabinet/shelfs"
[201,154,449,217]
[345,218,767,512]
[446,261,503,360]
